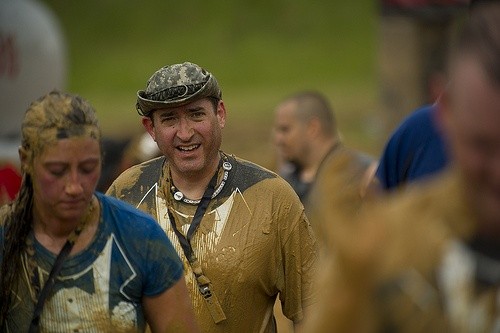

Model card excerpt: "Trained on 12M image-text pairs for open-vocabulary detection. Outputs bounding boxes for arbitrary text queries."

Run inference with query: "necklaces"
[170,152,233,204]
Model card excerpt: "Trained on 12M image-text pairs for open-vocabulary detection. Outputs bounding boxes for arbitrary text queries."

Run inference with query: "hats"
[136,62,224,115]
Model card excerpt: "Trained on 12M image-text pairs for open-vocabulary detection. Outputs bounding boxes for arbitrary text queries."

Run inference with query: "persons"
[306,0,500,333]
[272,88,377,333]
[0,88,199,333]
[105,62,322,333]
